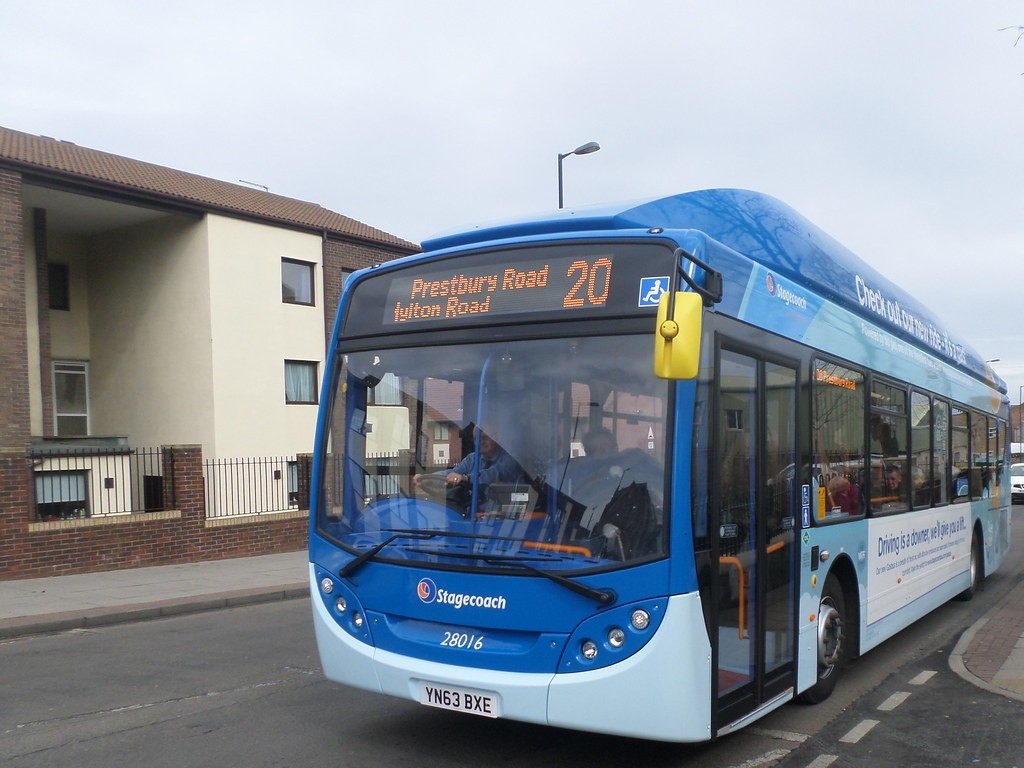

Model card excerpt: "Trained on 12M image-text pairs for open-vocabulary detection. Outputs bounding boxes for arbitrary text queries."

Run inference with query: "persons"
[880,465,906,509]
[413,424,535,517]
[558,427,664,511]
[911,466,940,506]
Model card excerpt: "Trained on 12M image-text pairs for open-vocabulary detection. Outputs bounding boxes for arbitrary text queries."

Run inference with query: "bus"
[306,186,1013,744]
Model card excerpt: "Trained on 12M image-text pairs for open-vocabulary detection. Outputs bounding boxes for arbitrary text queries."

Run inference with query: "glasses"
[472,433,497,441]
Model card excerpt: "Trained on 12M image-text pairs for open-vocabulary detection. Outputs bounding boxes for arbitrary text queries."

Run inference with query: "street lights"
[985,358,1000,367]
[556,141,601,208]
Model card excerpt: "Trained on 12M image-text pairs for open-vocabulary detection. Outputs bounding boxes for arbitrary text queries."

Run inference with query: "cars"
[1008,463,1024,504]
